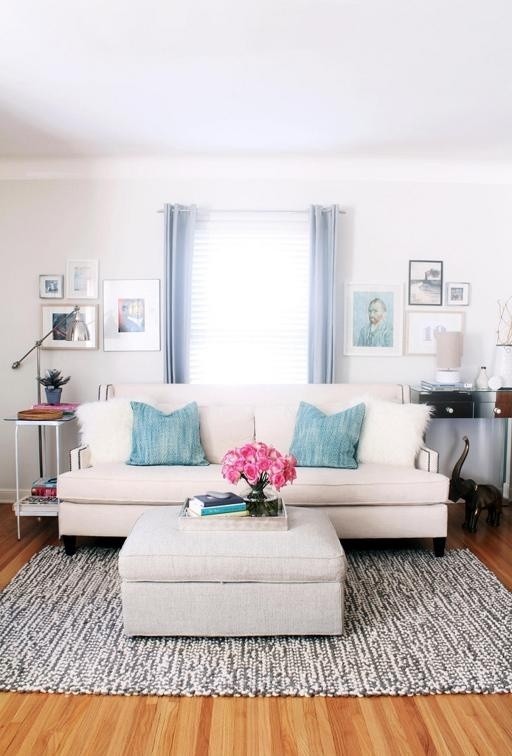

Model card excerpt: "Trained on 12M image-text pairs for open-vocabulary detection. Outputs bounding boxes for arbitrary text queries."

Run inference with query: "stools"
[117,509,345,639]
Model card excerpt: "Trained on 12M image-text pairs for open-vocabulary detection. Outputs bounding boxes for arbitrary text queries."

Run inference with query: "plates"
[17,409,63,421]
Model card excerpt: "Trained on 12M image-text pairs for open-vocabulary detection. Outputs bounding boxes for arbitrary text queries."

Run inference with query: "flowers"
[222,442,297,491]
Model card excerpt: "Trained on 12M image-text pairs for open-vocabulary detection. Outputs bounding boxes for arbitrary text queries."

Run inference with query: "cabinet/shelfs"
[13,416,75,541]
[408,385,511,509]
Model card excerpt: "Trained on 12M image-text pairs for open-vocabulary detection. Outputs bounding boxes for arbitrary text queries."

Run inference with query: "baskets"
[16,410,64,420]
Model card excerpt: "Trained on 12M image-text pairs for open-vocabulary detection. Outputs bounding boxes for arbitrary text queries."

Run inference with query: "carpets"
[0,546,512,700]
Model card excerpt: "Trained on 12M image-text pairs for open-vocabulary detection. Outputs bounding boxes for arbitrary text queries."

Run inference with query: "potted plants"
[38,369,71,404]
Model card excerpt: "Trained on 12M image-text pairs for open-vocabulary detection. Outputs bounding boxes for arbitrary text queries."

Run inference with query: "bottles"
[476,366,488,390]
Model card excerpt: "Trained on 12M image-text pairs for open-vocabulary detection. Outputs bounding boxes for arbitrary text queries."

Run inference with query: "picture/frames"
[37,258,162,351]
[340,259,471,358]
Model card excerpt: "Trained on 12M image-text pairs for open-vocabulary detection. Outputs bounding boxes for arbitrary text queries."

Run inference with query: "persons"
[356,298,393,346]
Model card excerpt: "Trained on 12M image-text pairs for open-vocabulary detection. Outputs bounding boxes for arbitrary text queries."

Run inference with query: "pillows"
[288,402,365,469]
[126,401,210,467]
[352,396,435,470]
[75,397,155,465]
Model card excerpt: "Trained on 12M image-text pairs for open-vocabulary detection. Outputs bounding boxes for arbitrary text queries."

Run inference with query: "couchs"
[57,383,447,558]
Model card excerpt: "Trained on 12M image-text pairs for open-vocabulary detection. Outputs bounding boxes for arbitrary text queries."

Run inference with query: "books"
[32,401,81,413]
[186,511,250,518]
[32,477,58,487]
[192,491,244,506]
[21,496,65,505]
[189,500,246,516]
[31,487,57,496]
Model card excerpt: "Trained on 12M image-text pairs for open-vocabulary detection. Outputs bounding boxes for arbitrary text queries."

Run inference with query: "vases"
[495,344,512,389]
[245,489,278,516]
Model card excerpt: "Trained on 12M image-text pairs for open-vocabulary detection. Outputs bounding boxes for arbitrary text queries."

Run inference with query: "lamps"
[435,331,462,385]
[12,305,90,478]
